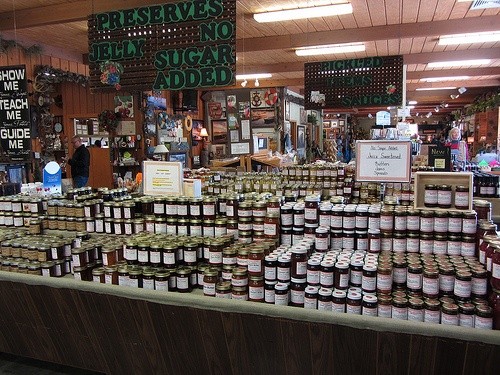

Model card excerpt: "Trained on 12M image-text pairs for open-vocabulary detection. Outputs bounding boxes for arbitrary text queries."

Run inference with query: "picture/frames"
[297,125,306,149]
[285,99,290,121]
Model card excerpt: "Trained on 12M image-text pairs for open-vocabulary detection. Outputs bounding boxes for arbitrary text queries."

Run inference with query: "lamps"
[251,2,500,69]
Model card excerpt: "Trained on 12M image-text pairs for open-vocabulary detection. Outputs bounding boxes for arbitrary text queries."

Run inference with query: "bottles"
[454,160,500,198]
[20,182,42,192]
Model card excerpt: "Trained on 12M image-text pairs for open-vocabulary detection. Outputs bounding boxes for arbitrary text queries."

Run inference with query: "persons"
[445,127,468,162]
[64,135,90,189]
[95,140,101,148]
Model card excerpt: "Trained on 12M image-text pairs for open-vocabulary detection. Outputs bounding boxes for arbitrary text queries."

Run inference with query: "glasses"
[71,140,79,144]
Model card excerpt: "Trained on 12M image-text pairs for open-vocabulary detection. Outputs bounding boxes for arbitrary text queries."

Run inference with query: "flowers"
[98,109,119,133]
